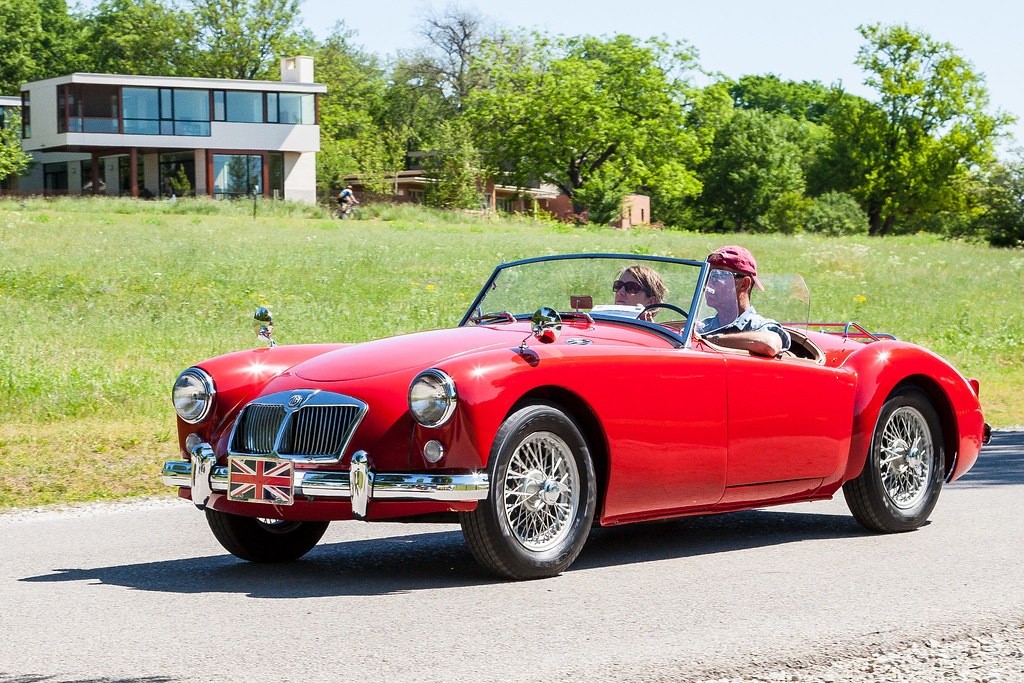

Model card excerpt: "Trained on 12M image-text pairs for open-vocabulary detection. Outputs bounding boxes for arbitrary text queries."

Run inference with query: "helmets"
[347,185,353,189]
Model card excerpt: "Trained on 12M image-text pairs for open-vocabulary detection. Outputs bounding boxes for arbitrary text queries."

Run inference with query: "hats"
[706,245,764,294]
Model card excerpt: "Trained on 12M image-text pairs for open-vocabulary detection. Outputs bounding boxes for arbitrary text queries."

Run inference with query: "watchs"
[712,335,719,345]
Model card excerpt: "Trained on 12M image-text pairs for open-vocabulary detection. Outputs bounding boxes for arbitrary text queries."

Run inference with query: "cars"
[159,248,994,584]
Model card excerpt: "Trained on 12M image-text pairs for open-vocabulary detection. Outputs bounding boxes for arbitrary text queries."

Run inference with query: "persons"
[337,185,359,219]
[612,264,665,323]
[640,245,791,357]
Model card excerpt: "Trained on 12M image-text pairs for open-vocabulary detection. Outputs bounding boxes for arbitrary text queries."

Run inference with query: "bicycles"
[331,201,362,221]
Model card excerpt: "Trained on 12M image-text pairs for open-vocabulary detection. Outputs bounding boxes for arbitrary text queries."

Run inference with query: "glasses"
[709,268,747,281]
[612,280,647,295]
